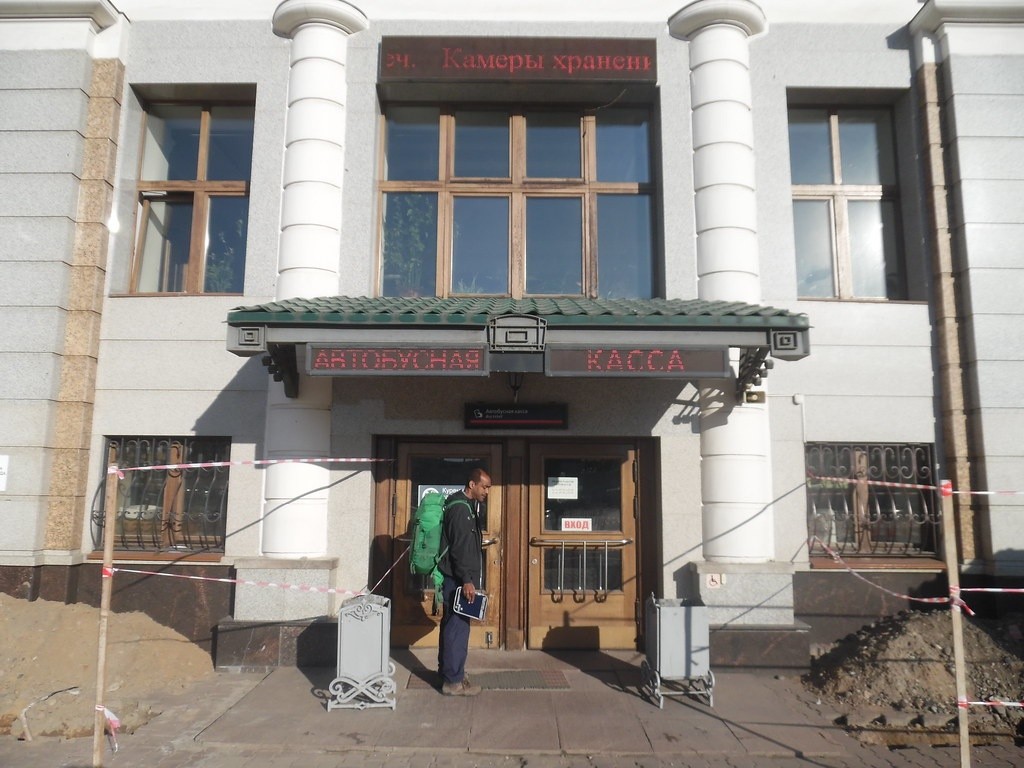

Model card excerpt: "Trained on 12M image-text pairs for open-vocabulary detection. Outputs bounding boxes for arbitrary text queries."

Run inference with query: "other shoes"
[442,681,481,696]
[437,669,470,684]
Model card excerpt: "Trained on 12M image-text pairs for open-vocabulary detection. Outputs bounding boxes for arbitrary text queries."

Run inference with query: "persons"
[438,468,492,696]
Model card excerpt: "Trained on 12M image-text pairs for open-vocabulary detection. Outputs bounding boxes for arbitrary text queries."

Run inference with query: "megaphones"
[736,390,765,404]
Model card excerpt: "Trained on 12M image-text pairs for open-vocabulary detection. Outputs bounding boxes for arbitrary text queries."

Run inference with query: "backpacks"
[408,492,475,576]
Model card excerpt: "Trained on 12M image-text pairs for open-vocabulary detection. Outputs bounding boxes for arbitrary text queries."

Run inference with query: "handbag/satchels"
[453,587,488,621]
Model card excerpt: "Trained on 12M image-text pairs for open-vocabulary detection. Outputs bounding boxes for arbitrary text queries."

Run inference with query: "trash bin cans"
[639,591,716,709]
[329,594,396,715]
